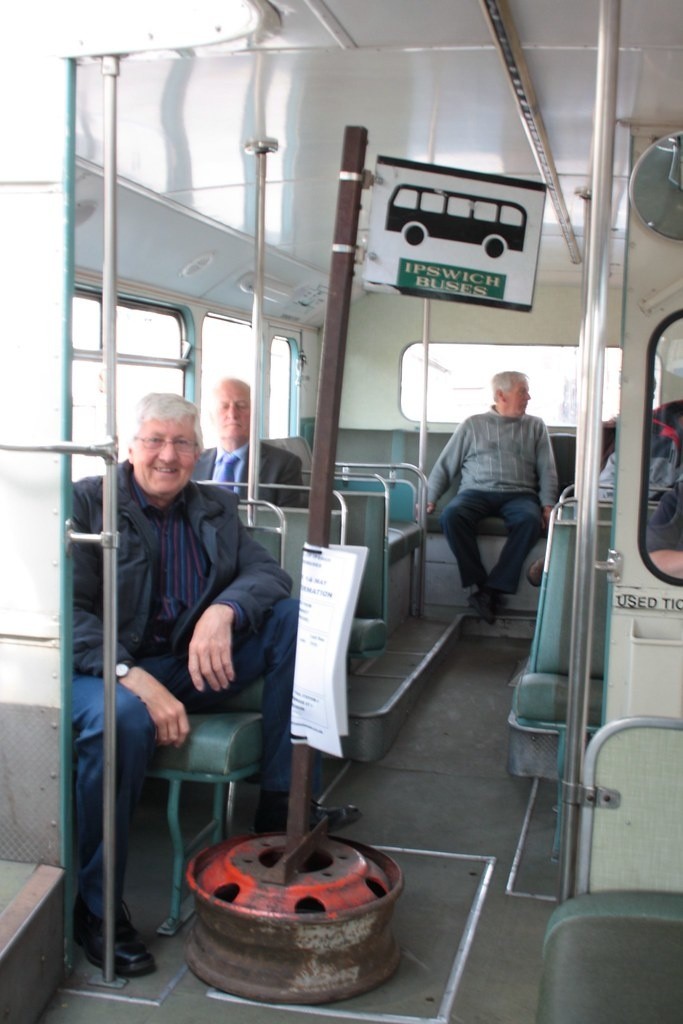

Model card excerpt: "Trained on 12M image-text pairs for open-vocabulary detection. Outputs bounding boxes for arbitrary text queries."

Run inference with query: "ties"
[218,455,240,493]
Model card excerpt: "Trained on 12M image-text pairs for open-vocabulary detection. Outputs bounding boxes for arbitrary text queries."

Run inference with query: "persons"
[71,394,365,980]
[526,397,682,589]
[415,371,558,627]
[184,379,303,514]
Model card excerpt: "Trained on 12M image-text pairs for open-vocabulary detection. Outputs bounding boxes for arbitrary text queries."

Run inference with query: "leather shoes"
[73,896,153,976]
[256,788,362,835]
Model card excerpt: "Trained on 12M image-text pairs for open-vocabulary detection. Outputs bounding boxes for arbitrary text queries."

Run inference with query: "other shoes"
[467,586,508,625]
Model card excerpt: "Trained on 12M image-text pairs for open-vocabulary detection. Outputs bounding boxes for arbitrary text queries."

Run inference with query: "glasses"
[136,436,198,453]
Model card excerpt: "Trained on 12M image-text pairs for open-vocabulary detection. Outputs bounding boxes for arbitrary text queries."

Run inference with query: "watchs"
[116,662,135,683]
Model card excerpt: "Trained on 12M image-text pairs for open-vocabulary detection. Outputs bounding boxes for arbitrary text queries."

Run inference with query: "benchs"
[72,424,682,1024]
